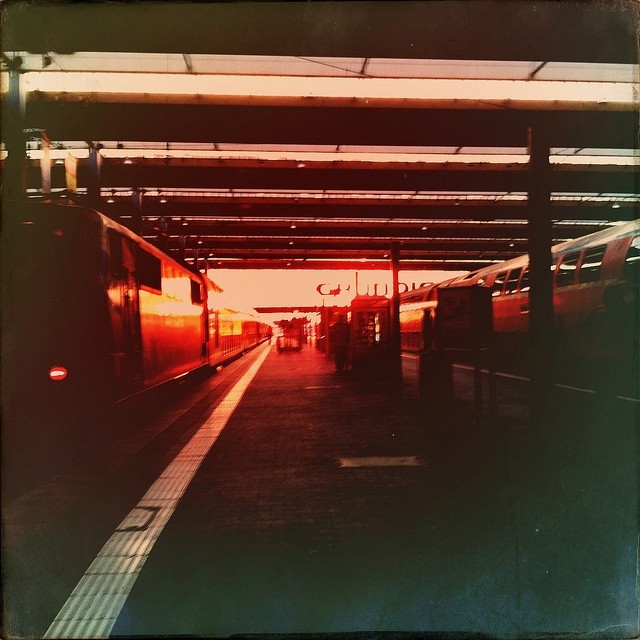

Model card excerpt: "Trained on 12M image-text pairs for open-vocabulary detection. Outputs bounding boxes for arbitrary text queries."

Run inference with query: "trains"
[397,219,640,355]
[0,189,274,406]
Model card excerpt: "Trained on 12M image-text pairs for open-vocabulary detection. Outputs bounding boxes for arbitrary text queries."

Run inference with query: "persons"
[330,314,350,374]
[266,326,274,344]
